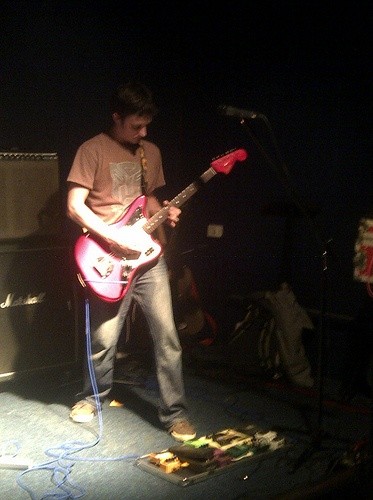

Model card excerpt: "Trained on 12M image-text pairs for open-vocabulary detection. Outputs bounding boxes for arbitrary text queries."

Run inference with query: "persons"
[65,83,199,441]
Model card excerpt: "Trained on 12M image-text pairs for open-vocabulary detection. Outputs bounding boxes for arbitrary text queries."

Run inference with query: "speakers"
[0,151,86,375]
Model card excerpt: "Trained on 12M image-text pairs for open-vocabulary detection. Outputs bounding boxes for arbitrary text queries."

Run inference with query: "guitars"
[73,148,247,302]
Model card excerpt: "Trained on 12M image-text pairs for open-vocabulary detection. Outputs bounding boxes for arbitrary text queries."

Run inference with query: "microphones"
[218,104,264,119]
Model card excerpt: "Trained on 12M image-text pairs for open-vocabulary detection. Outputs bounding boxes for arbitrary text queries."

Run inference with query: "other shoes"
[165,418,196,443]
[69,395,104,424]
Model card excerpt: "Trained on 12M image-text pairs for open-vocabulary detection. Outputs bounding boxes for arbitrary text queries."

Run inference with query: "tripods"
[239,119,354,475]
